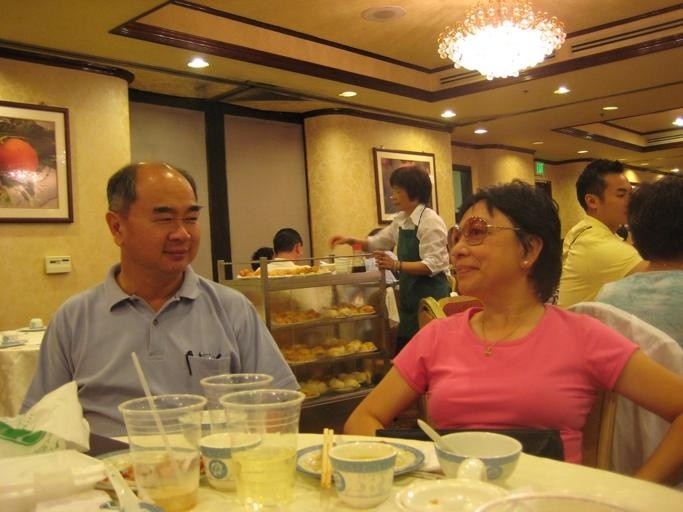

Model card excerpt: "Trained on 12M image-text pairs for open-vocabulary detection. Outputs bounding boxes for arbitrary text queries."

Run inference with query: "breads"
[321,303,375,319]
[280,344,314,362]
[297,378,328,397]
[239,265,319,277]
[314,334,377,358]
[270,308,320,325]
[329,369,372,393]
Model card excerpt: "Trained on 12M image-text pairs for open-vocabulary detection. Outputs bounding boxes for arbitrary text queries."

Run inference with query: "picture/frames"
[0,100,74,223]
[374,148,438,224]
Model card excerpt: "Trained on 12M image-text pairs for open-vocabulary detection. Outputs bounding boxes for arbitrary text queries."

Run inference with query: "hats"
[445,215,524,251]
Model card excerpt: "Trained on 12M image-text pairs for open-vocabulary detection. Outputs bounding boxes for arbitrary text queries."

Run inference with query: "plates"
[296,439,425,478]
[1,340,28,347]
[477,494,625,512]
[395,481,508,511]
[95,448,171,492]
[17,326,48,332]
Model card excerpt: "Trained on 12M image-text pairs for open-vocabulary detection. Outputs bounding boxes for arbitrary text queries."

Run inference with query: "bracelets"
[350,237,355,244]
[394,261,401,272]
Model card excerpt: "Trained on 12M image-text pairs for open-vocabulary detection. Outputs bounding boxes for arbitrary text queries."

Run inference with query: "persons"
[329,166,451,357]
[20,161,302,448]
[361,229,399,285]
[251,247,274,270]
[342,179,683,485]
[594,175,683,346]
[550,160,651,310]
[255,228,312,272]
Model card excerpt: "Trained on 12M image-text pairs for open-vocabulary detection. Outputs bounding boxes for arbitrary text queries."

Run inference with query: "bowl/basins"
[334,257,350,272]
[433,430,524,486]
[319,264,335,272]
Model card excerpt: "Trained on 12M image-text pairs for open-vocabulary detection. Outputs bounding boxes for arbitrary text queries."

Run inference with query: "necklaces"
[482,303,540,357]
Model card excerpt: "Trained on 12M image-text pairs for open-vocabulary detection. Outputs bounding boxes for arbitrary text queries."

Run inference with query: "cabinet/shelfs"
[216,252,388,433]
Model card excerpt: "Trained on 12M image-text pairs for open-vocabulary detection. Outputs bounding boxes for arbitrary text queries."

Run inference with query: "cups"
[329,442,398,508]
[200,434,262,491]
[201,373,274,437]
[220,389,305,507]
[30,319,44,327]
[0,331,16,343]
[119,393,207,511]
[179,410,246,448]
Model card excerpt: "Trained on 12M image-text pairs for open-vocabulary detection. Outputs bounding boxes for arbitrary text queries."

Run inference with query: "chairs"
[438,294,487,315]
[582,389,617,471]
[567,302,682,490]
[418,297,447,430]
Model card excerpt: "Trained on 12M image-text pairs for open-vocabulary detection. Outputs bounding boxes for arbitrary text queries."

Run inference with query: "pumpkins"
[0,136,39,177]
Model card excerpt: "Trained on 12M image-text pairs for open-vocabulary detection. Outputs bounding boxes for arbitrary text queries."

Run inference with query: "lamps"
[437,0,567,81]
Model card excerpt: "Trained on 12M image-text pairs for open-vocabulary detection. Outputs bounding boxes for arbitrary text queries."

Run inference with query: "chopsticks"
[320,427,334,488]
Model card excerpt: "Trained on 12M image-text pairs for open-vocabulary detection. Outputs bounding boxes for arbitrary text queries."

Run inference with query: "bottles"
[351,243,366,272]
